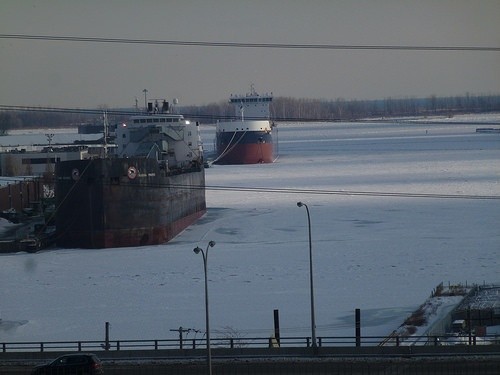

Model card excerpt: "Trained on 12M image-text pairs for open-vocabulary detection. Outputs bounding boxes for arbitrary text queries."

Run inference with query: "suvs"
[33,354,104,375]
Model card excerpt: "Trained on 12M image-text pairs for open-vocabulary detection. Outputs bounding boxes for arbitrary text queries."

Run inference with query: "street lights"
[193,239,219,375]
[296,201,320,352]
